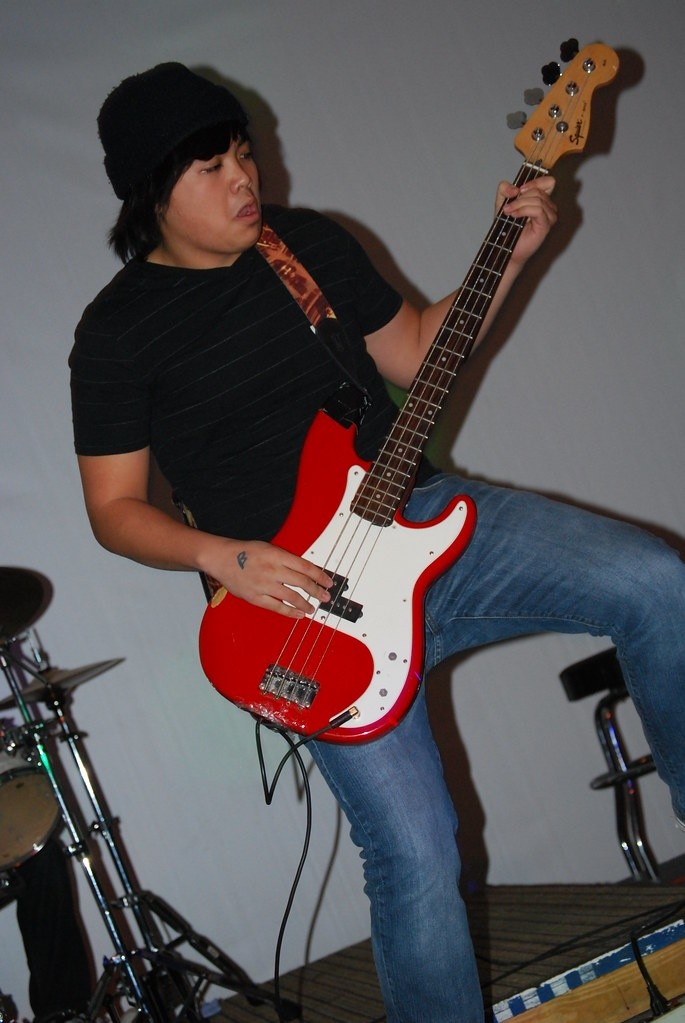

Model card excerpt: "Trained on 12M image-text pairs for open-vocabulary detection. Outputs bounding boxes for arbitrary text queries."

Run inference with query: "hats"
[96,61,249,200]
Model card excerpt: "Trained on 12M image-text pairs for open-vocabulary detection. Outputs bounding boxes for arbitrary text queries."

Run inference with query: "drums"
[0,732,58,872]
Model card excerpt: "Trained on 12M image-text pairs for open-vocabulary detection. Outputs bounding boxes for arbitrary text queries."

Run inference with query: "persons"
[69,61,685,1023]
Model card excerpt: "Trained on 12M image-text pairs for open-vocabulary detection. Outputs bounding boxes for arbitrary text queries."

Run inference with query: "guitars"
[196,36,626,745]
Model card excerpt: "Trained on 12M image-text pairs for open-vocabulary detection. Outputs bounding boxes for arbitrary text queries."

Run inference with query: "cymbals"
[0,656,127,709]
[0,565,55,647]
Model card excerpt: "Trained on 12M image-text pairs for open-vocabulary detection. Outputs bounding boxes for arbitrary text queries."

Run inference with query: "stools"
[559,642,659,883]
